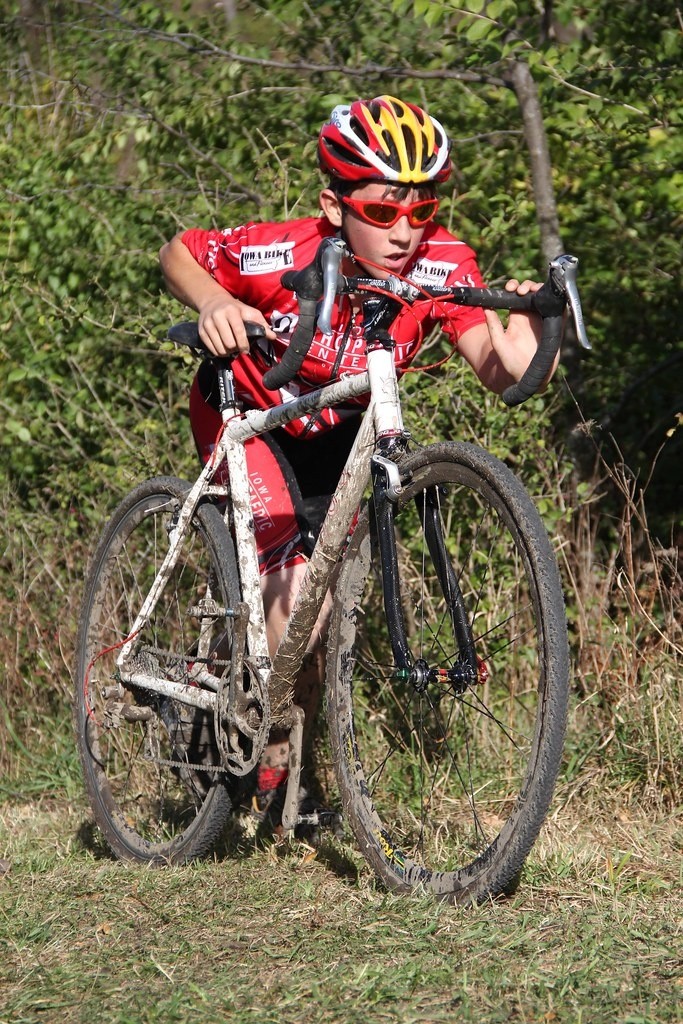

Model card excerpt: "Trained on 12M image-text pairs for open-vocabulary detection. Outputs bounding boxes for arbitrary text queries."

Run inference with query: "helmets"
[318,95,453,187]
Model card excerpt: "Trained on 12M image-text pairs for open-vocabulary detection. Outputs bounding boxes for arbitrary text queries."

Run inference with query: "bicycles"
[74,236,593,911]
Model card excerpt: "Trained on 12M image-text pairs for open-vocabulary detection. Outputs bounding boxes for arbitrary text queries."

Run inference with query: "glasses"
[337,193,439,228]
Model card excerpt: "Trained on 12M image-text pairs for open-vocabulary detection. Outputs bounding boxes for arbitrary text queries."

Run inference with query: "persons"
[156,93,569,867]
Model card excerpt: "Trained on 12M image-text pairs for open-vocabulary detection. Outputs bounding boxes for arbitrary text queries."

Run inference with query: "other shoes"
[251,792,333,826]
